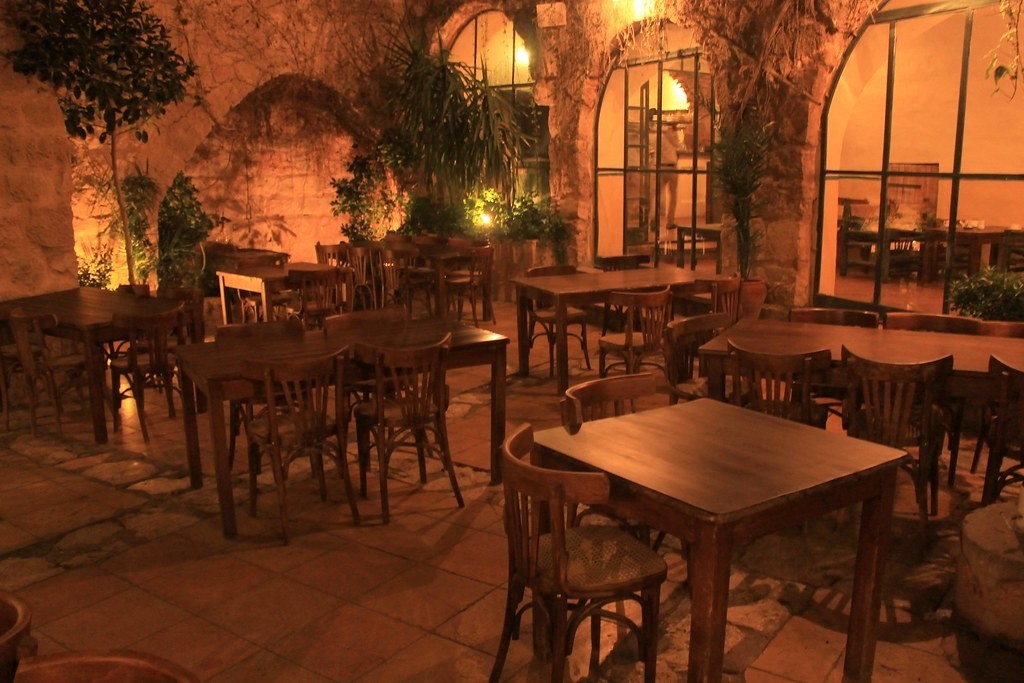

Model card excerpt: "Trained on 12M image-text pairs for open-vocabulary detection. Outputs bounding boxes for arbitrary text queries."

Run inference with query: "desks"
[528,398,908,683]
[336,239,473,318]
[678,224,723,268]
[217,263,355,325]
[697,317,1024,403]
[511,266,717,395]
[0,288,206,443]
[924,225,1009,289]
[170,308,507,538]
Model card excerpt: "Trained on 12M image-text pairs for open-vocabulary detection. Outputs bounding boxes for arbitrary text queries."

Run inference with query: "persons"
[650,122,679,231]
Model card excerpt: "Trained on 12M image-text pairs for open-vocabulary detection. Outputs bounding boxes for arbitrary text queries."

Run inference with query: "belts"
[661,164,675,166]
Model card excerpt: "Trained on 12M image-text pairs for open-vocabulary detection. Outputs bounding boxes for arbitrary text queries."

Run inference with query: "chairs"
[836,196,1024,285]
[0,591,199,683]
[4,231,499,548]
[486,253,1024,683]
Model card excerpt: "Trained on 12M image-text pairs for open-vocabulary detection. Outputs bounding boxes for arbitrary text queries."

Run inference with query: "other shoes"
[665,222,677,229]
[650,221,657,232]
